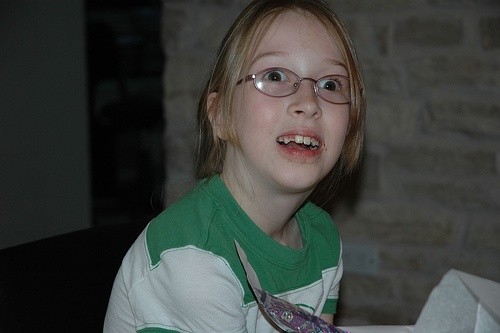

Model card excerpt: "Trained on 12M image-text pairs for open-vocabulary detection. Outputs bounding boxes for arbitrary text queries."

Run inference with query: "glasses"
[212,67,353,104]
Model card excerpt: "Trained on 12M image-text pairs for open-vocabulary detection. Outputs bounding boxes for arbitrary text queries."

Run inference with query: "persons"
[103,0,369,333]
